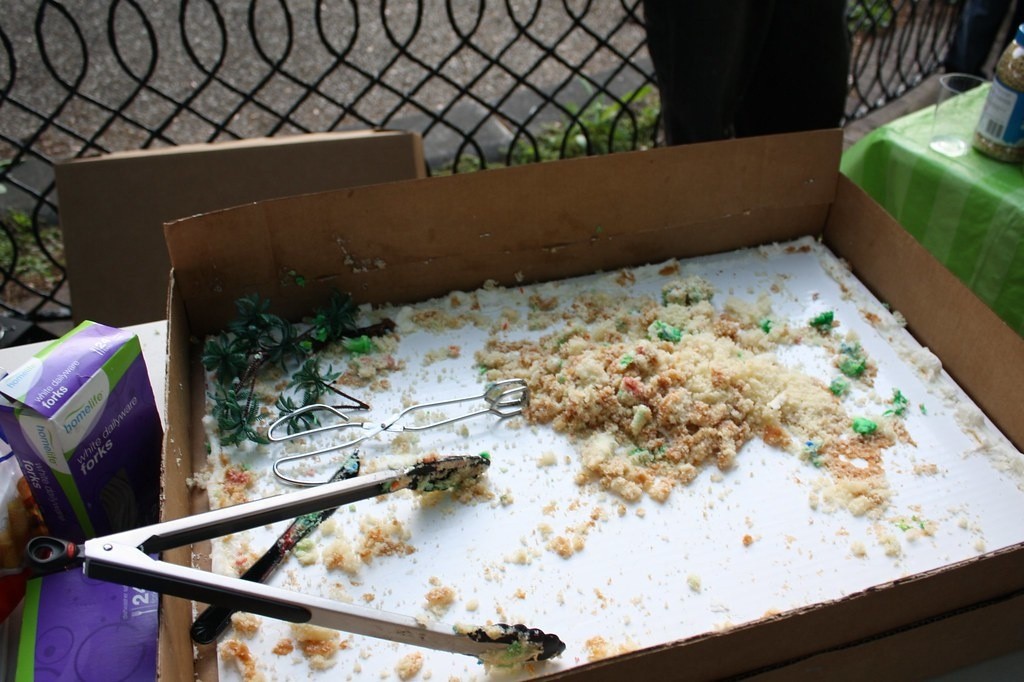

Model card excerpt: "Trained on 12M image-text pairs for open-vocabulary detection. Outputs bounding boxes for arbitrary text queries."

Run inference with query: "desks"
[840,82,1024,337]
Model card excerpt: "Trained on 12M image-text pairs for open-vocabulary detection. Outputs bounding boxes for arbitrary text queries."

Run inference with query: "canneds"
[971,23,1024,162]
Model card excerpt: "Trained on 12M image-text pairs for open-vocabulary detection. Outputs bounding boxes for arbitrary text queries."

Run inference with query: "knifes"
[190,449,360,646]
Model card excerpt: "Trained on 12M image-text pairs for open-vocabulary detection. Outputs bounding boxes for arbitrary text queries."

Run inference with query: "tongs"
[22,455,566,665]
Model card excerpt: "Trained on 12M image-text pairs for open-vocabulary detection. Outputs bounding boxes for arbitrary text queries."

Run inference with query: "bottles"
[971,23,1024,162]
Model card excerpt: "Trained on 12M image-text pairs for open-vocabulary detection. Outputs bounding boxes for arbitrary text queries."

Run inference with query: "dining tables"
[0,321,167,682]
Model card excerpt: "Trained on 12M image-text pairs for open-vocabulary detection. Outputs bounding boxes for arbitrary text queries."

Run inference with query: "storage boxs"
[0,319,163,543]
[154,129,1024,682]
[55,130,425,326]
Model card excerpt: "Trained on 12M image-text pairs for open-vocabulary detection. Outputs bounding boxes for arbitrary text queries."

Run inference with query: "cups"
[927,73,991,159]
